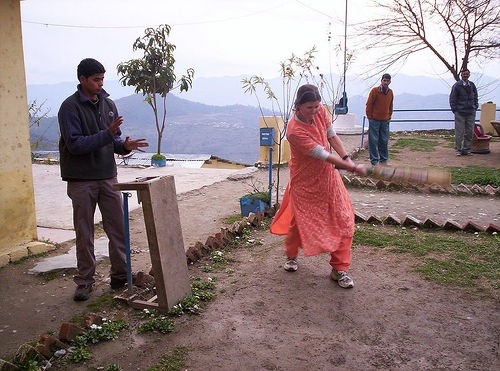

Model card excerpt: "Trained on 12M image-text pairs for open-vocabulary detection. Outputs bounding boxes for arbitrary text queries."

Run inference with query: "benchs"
[112,175,191,311]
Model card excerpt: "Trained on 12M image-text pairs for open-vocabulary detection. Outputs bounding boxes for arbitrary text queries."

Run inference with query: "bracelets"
[343,155,348,160]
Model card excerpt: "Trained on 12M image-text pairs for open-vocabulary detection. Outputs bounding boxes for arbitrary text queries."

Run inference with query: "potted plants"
[151,154,166,167]
[239,192,271,217]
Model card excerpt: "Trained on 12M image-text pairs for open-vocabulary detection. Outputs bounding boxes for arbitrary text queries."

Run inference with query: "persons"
[339,92,347,108]
[271,84,367,288]
[449,68,479,156]
[366,73,393,167]
[58,58,149,301]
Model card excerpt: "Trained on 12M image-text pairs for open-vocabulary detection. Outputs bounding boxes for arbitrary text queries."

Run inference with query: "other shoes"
[73,283,93,300]
[111,274,136,289]
[456,151,473,156]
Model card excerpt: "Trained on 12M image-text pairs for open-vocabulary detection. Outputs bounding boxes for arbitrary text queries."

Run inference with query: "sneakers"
[284,256,298,271]
[329,266,354,288]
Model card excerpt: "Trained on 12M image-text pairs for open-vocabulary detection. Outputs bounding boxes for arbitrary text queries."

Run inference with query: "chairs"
[470,123,492,154]
